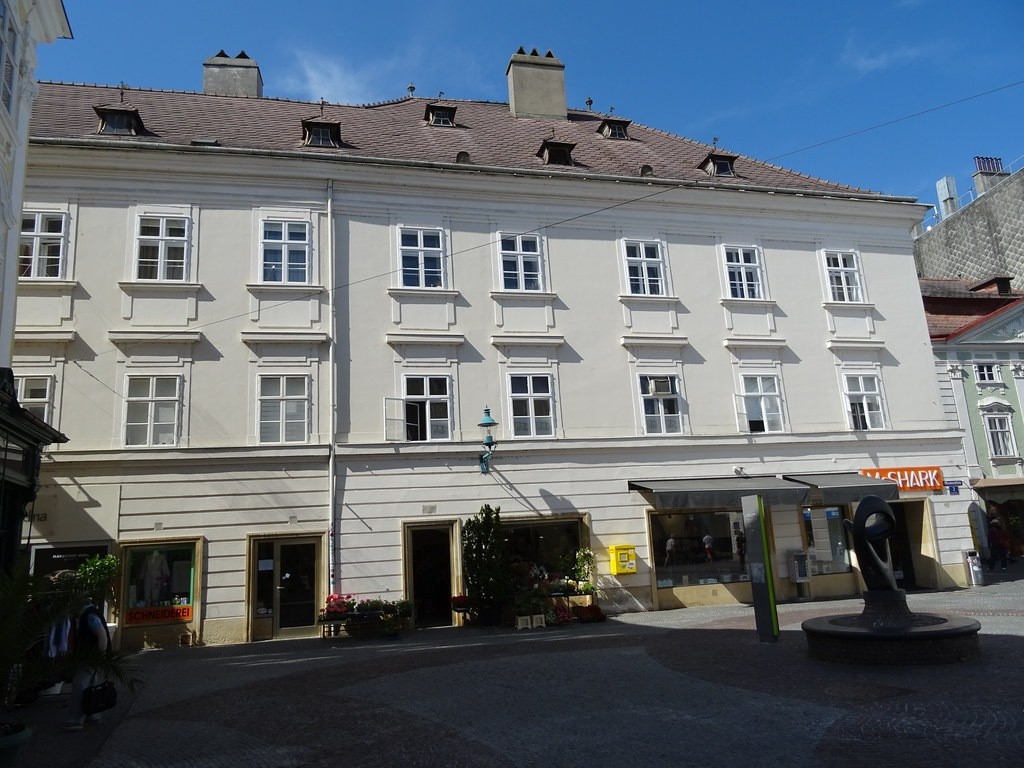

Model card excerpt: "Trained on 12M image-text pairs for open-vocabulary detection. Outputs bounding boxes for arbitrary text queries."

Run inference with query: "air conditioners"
[650,380,671,394]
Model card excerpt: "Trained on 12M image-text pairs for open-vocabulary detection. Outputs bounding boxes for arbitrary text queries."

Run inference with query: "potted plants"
[0,557,149,768]
[512,589,553,630]
[461,503,515,627]
[582,583,596,594]
[399,600,412,617]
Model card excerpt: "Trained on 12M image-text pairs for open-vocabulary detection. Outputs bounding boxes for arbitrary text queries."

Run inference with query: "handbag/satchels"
[83,665,118,715]
[999,528,1009,547]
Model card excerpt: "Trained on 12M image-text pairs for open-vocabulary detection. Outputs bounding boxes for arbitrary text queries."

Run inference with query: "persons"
[60,595,112,729]
[139,549,170,607]
[703,532,713,562]
[664,534,675,568]
[736,532,745,570]
[986,519,1008,571]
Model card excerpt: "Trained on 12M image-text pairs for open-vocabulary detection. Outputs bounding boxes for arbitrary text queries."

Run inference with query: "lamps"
[476,404,500,473]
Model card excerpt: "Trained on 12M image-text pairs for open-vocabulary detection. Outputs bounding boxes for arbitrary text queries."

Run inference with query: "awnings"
[785,474,900,506]
[630,477,810,509]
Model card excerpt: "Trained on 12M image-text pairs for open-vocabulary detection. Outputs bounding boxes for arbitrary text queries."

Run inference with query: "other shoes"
[82,718,102,726]
[987,567,993,571]
[1003,568,1006,571]
[59,721,84,731]
[1009,559,1018,564]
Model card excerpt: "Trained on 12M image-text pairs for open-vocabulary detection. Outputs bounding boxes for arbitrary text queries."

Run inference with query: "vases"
[328,616,346,620]
[578,580,588,590]
[453,602,470,608]
[346,619,400,637]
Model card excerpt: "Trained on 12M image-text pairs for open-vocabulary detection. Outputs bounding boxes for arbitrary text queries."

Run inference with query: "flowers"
[546,571,578,591]
[449,595,472,603]
[546,605,606,625]
[318,593,398,622]
[572,547,596,581]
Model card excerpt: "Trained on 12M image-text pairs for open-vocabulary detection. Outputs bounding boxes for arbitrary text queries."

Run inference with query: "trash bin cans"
[962,548,985,589]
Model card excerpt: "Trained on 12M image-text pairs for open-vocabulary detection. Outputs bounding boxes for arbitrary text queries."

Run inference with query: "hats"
[989,519,999,523]
